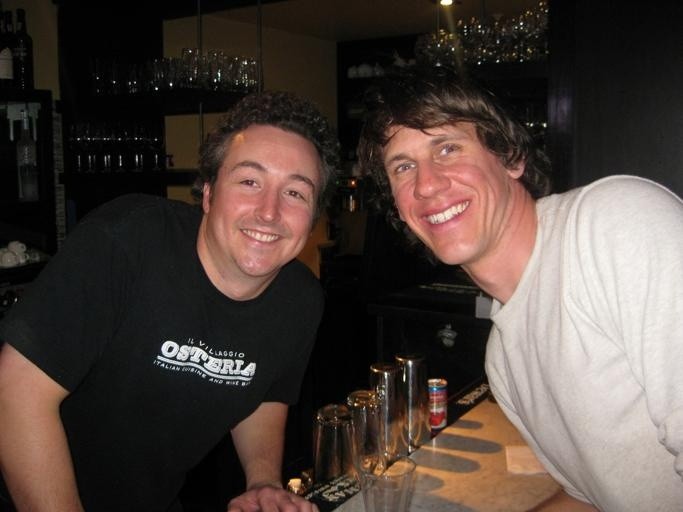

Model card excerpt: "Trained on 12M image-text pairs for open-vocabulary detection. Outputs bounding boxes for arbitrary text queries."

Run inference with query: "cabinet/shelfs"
[53,1,259,186]
[337,28,575,235]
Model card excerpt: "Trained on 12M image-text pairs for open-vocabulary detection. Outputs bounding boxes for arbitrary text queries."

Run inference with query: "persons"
[0,89,341,512]
[354,63,683,512]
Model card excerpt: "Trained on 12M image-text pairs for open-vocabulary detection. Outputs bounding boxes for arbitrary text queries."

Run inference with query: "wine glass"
[116,48,261,92]
[424,1,557,79]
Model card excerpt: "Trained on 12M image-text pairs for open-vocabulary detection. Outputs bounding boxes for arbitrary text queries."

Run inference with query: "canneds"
[426,378,448,430]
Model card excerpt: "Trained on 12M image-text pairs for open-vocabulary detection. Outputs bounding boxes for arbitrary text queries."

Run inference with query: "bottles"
[15,110,42,207]
[71,136,162,169]
[0,12,34,102]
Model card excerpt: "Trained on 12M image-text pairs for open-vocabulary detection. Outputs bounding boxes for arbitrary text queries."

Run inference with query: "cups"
[313,349,426,512]
